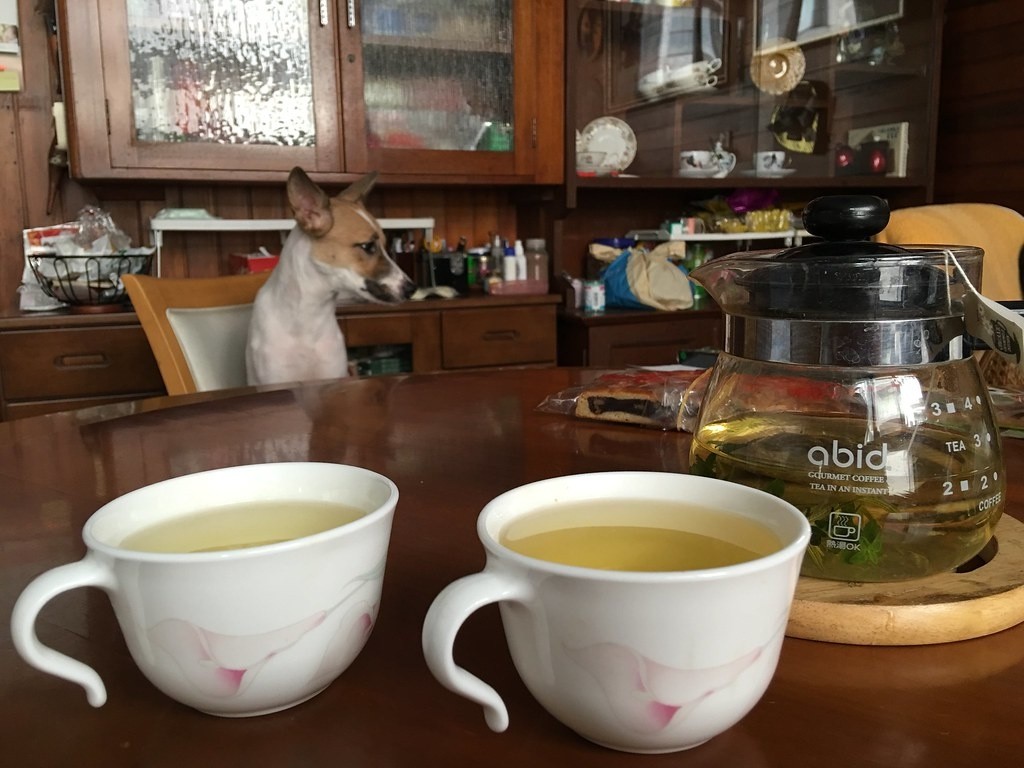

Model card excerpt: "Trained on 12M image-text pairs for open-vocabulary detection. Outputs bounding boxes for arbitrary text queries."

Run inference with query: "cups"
[575,151,607,166]
[678,150,716,169]
[715,142,737,178]
[8,459,401,717]
[634,57,721,100]
[681,216,707,233]
[752,151,793,169]
[419,468,812,754]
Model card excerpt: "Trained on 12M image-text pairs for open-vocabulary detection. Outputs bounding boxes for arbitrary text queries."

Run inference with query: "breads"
[577,370,850,433]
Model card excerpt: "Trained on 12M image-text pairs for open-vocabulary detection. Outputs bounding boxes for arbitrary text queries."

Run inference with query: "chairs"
[121,272,271,396]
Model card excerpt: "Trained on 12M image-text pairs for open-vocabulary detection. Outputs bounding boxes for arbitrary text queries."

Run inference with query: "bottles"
[392,230,417,284]
[465,229,549,291]
[439,237,466,253]
[690,243,707,299]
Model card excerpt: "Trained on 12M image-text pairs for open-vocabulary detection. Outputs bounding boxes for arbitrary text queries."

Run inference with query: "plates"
[581,115,638,174]
[576,166,613,176]
[677,167,719,177]
[740,169,797,178]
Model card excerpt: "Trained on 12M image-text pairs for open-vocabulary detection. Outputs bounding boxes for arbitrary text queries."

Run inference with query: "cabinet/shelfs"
[0,296,562,423]
[547,0,944,368]
[59,1,565,182]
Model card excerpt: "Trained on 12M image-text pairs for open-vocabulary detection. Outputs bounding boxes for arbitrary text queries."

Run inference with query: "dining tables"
[0,368,1024,768]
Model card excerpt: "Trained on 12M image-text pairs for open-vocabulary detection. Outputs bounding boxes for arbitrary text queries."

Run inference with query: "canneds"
[584,278,605,313]
[467,248,492,289]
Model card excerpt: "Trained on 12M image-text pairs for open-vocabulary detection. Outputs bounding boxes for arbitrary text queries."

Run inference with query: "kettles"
[688,190,1007,583]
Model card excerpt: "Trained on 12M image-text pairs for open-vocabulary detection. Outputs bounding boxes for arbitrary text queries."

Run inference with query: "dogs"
[245,165,418,389]
